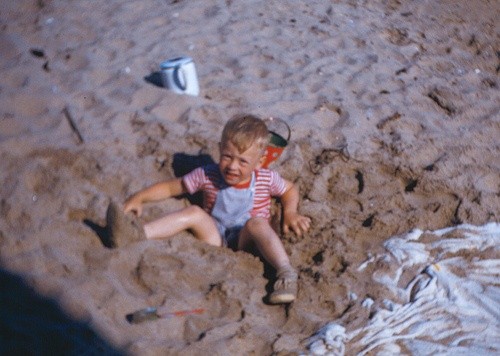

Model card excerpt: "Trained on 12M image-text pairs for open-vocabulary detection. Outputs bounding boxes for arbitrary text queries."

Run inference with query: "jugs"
[159,55,201,96]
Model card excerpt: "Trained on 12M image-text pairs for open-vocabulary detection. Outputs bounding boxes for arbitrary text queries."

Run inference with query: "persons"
[106,113,311,305]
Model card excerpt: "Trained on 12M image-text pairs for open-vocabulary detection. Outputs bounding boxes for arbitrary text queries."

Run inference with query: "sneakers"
[105,196,139,248]
[270,266,298,303]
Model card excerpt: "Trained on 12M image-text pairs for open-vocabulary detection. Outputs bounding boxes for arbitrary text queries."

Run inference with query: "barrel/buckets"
[261,117,292,170]
